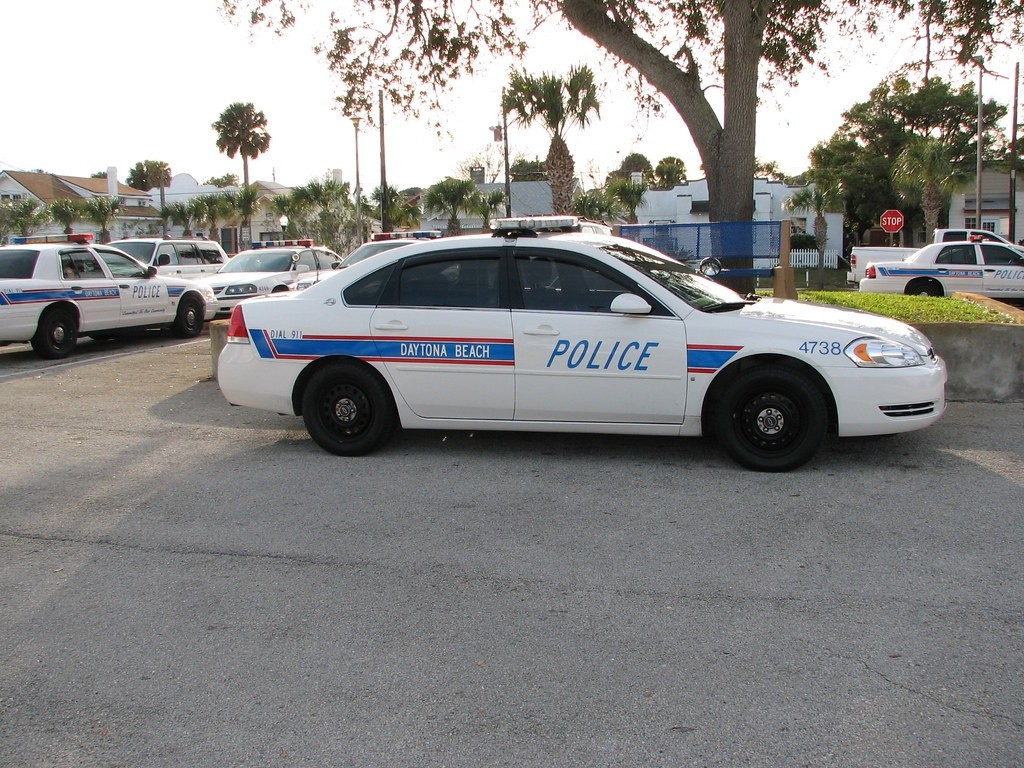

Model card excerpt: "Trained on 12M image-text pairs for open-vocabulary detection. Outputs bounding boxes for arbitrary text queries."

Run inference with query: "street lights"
[349,113,364,246]
[973,52,985,231]
[280,215,289,237]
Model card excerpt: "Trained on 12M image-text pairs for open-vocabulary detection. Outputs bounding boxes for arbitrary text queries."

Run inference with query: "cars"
[293,231,442,292]
[0,233,217,360]
[192,239,344,314]
[218,214,949,476]
[859,235,1024,298]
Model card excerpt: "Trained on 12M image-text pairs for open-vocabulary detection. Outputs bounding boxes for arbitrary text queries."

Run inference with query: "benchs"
[611,219,798,300]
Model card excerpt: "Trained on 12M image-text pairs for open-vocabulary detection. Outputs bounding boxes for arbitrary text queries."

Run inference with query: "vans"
[105,234,230,280]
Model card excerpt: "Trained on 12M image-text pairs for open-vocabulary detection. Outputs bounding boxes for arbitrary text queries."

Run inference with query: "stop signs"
[880,209,904,234]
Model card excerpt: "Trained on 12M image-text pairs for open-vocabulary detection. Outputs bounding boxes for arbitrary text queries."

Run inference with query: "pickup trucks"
[846,228,1013,284]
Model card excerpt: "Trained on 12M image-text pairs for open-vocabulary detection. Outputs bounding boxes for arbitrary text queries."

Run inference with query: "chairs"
[418,272,462,306]
[526,255,566,309]
